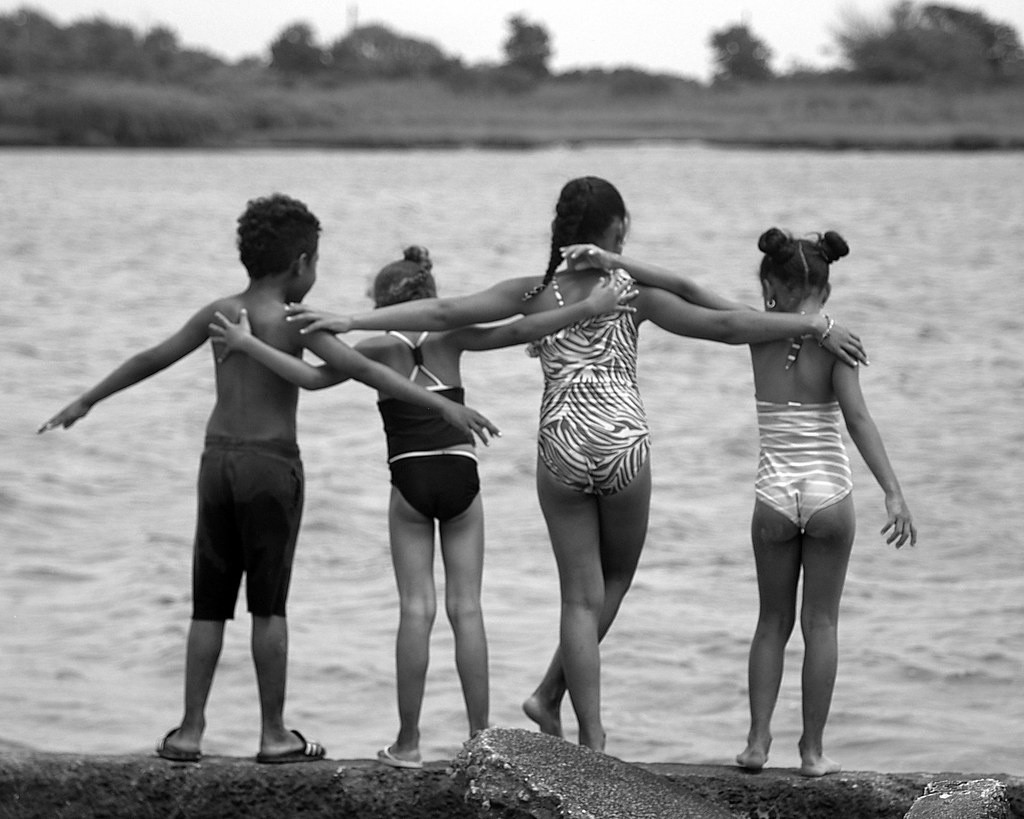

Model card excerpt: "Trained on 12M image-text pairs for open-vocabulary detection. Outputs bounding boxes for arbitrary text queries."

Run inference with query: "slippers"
[377,745,423,768]
[157,726,202,760]
[256,729,325,762]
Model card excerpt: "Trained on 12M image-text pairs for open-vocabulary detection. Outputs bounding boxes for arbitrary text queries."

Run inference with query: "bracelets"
[821,318,834,338]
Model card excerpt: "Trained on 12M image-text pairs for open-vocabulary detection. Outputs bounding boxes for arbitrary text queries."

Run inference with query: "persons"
[207,245,639,769]
[559,226,918,776]
[282,175,869,752]
[37,193,502,764]
